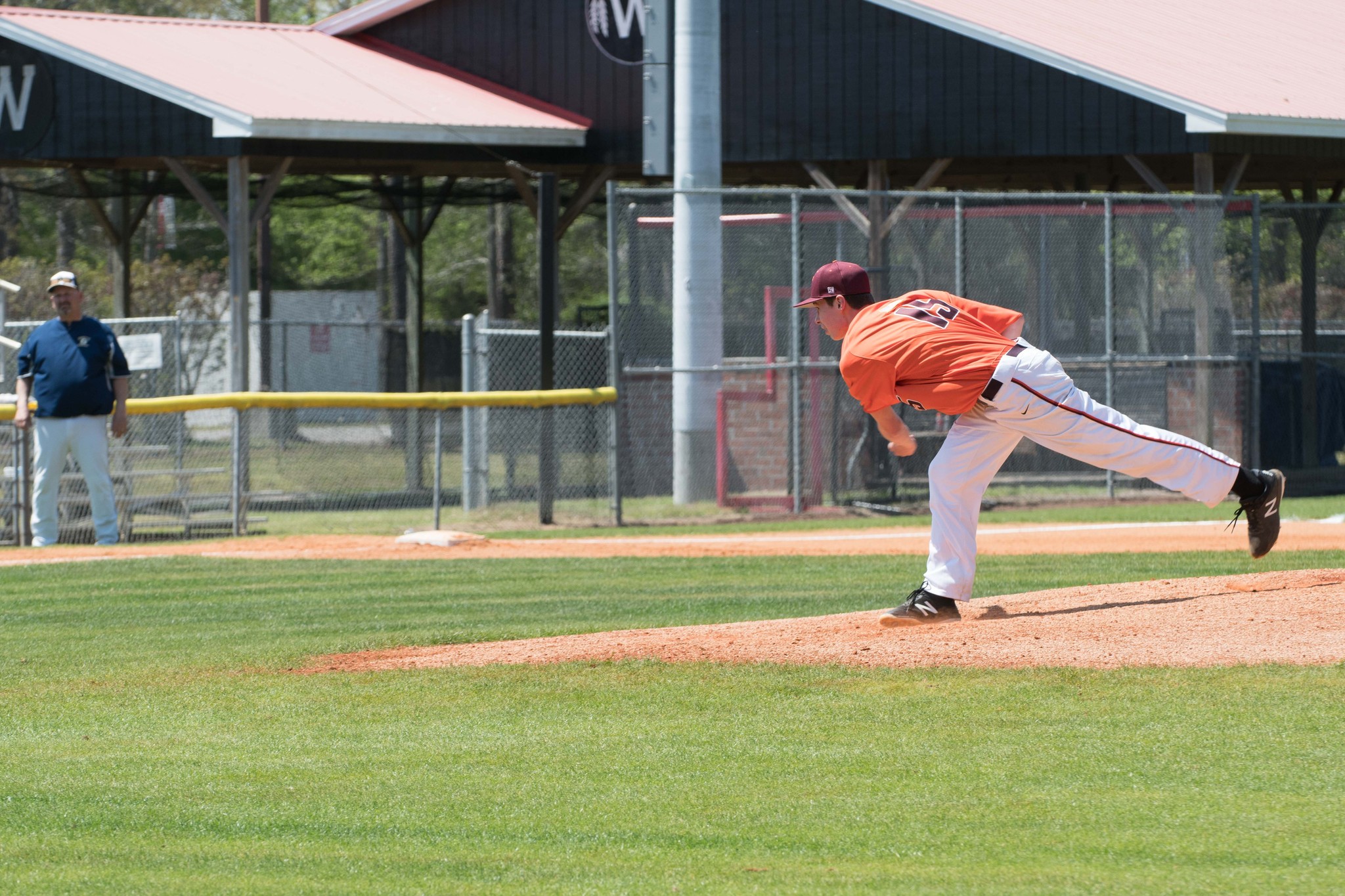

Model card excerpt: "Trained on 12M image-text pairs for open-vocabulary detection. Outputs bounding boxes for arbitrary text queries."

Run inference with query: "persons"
[14,271,132,547]
[793,260,1285,625]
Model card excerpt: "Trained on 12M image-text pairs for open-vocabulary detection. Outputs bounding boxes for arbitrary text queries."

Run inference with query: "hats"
[793,260,870,308]
[46,271,78,293]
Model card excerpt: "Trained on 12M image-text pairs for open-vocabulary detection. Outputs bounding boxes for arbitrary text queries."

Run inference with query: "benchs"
[0,394,268,542]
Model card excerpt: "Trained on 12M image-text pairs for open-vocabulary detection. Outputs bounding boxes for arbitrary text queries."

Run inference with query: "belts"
[977,344,1027,405]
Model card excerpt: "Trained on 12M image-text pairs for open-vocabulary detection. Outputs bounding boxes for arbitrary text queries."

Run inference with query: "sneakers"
[1223,469,1285,558]
[879,581,961,628]
